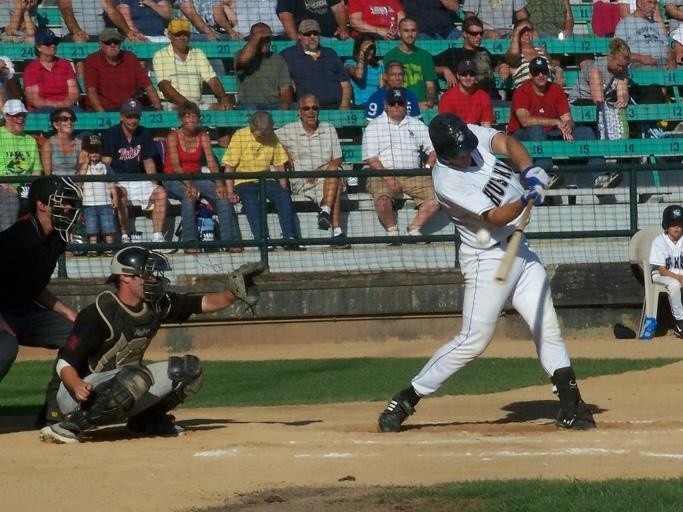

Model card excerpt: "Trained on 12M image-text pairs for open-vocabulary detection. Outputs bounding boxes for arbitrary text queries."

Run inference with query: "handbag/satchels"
[602,89,638,103]
[597,101,629,141]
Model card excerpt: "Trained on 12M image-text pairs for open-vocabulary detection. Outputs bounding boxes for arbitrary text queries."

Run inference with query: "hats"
[3,100,28,115]
[299,19,320,35]
[121,98,142,117]
[529,58,547,71]
[385,86,407,105]
[168,19,192,34]
[458,58,478,75]
[81,135,101,153]
[35,30,62,44]
[99,28,122,43]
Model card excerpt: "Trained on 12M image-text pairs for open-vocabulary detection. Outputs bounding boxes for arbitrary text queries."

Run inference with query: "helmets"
[110,246,172,303]
[428,113,478,164]
[28,176,83,244]
[662,205,683,228]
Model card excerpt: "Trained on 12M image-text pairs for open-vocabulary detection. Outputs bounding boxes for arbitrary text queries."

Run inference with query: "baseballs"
[477,229,490,244]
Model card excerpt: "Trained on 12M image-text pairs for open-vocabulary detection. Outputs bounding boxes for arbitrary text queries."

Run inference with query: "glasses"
[58,116,75,122]
[464,32,484,36]
[302,106,319,110]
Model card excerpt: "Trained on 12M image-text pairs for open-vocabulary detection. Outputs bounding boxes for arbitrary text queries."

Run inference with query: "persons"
[0,0,244,253]
[222,109,308,252]
[379,112,597,432]
[0,176,97,429]
[649,206,683,339]
[380,0,682,188]
[358,88,440,247]
[272,93,352,250]
[39,245,264,444]
[227,1,406,143]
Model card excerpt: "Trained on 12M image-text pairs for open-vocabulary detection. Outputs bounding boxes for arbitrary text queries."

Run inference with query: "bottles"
[388,16,396,31]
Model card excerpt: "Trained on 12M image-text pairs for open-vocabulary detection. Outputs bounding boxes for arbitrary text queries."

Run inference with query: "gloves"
[519,166,549,205]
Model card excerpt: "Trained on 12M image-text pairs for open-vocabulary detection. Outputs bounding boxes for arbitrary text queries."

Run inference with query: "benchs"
[1,1,683,210]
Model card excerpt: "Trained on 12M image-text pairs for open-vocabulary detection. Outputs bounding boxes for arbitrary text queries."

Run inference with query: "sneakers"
[556,409,596,430]
[593,173,623,188]
[407,227,430,244]
[331,234,351,249]
[318,213,332,230]
[378,392,415,432]
[386,226,402,246]
[38,424,82,444]
[546,175,560,189]
[673,320,683,339]
[129,420,184,438]
[72,233,200,256]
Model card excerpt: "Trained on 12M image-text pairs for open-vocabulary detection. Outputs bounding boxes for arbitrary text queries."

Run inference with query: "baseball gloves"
[228,263,269,305]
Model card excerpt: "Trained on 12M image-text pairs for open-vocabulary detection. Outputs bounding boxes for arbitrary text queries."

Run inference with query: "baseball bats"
[495,195,535,285]
